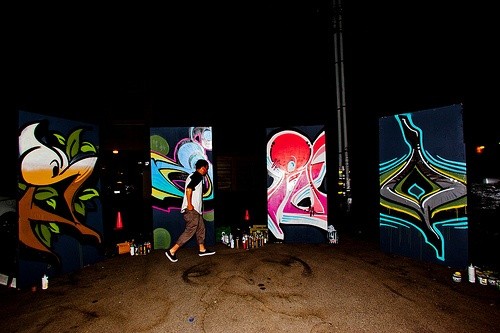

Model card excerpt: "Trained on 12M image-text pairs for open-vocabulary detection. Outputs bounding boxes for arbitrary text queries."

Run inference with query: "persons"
[164,159,216,263]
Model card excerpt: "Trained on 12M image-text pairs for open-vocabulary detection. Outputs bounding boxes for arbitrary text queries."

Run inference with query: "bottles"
[452,263,500,287]
[42,274,48,290]
[130,239,151,256]
[221,230,269,250]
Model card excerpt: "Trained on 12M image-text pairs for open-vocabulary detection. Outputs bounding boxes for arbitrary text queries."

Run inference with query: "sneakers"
[165,250,178,263]
[199,249,216,257]
